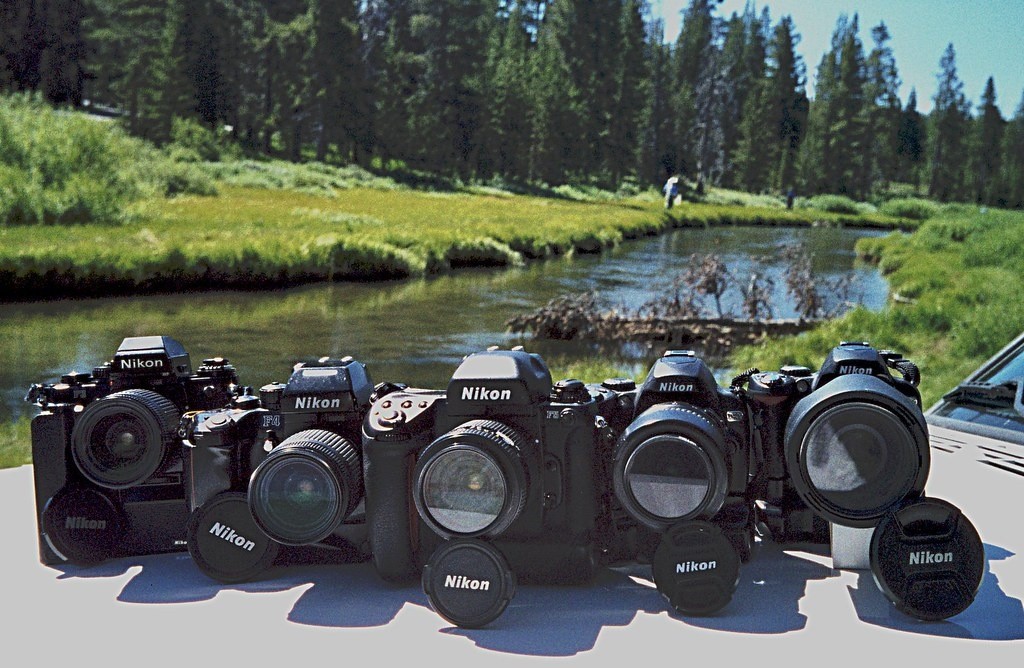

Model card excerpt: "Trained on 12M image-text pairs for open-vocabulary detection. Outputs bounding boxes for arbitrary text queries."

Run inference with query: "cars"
[0,328,1024,664]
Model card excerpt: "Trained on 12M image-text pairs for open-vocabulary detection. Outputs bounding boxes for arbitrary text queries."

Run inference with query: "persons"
[787,188,794,208]
[663,178,678,209]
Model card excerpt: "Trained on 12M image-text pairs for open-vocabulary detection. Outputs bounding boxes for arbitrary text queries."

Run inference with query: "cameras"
[22,334,987,622]
